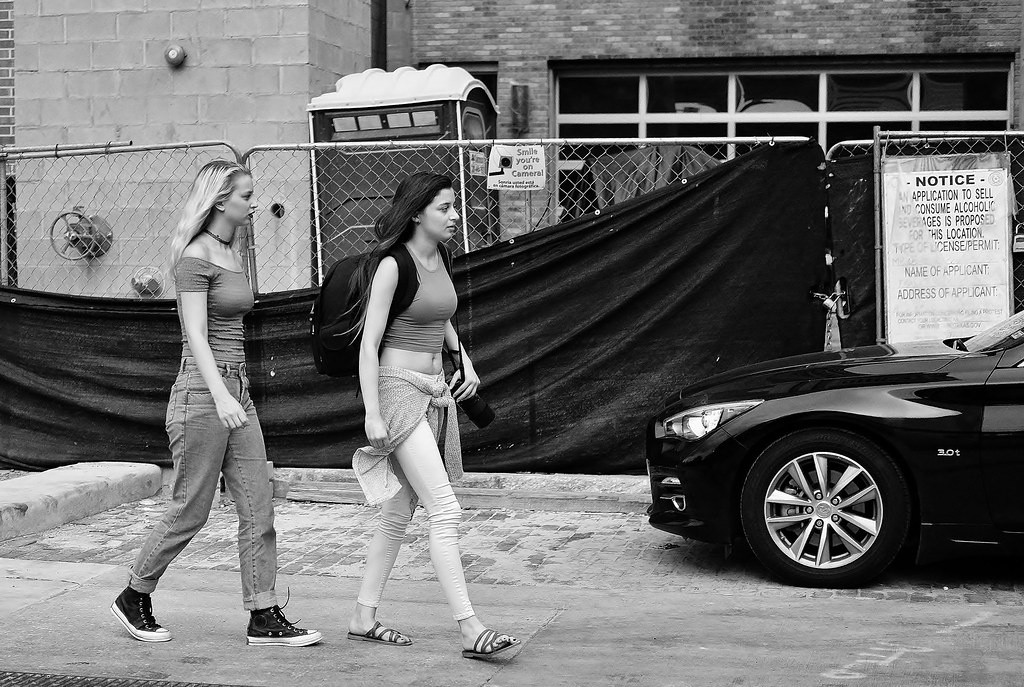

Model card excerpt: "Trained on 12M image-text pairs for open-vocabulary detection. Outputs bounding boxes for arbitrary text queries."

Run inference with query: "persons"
[110,159,325,646]
[347,174,522,658]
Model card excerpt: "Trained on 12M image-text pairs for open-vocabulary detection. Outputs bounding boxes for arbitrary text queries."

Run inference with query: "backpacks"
[309,241,452,379]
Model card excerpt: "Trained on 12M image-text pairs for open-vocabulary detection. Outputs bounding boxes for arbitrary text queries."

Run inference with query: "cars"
[643,309,1024,589]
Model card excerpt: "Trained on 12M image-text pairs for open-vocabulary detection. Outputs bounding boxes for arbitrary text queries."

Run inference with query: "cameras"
[446,378,496,429]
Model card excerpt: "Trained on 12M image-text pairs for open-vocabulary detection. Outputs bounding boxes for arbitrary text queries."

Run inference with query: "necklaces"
[204,229,231,245]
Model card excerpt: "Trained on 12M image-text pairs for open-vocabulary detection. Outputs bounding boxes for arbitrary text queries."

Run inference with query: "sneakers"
[110,586,172,642]
[246,586,324,647]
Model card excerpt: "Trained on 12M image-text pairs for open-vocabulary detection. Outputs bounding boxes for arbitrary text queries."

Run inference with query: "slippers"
[347,621,413,646]
[462,628,521,660]
